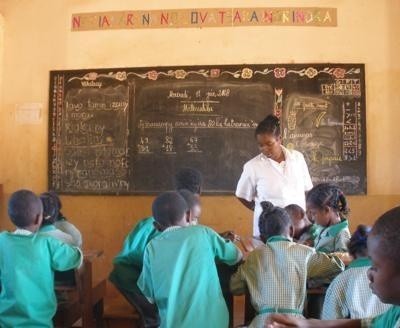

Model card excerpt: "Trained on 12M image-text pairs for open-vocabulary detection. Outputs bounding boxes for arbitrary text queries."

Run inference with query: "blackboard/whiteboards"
[48,64,367,196]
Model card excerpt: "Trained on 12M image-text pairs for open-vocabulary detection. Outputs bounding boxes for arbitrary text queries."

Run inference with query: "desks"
[230,286,327,328]
[53,249,107,328]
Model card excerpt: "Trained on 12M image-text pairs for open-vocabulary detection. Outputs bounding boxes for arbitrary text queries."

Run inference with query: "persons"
[284,204,313,239]
[263,207,400,328]
[38,195,73,242]
[141,189,238,320]
[228,201,345,328]
[136,191,243,328]
[303,184,352,252]
[321,224,393,320]
[107,170,203,327]
[40,192,82,246]
[0,190,83,328]
[235,114,315,237]
[298,190,314,245]
